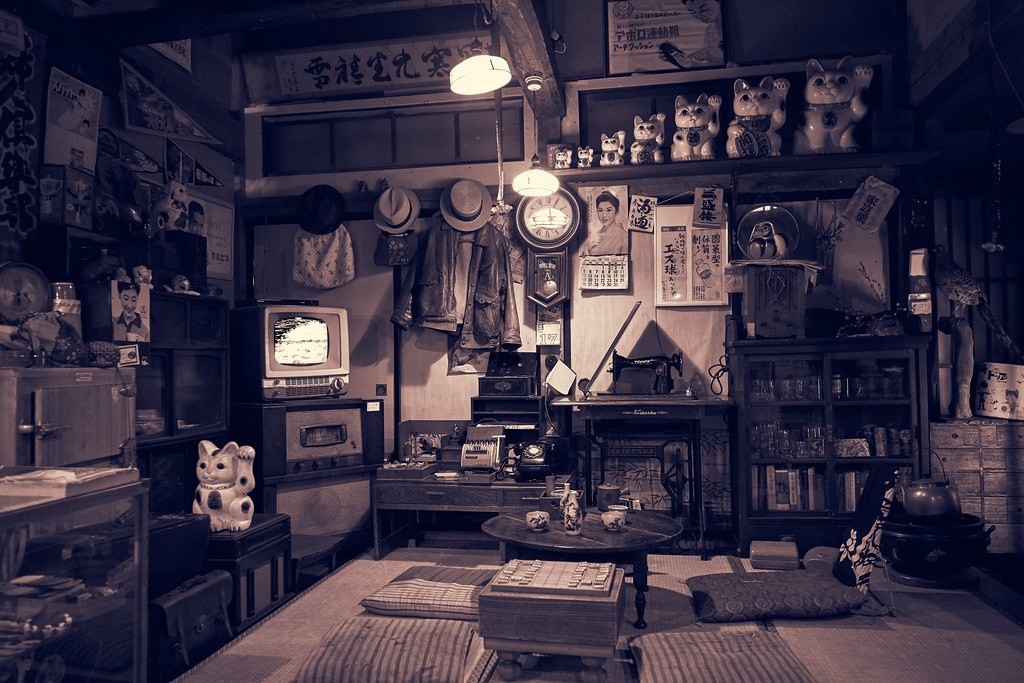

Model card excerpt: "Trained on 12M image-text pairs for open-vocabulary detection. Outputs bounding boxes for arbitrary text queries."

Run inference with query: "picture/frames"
[654,203,726,306]
[268,30,518,100]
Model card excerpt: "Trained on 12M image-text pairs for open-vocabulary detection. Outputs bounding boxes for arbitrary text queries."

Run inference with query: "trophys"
[39,173,64,213]
[69,176,92,224]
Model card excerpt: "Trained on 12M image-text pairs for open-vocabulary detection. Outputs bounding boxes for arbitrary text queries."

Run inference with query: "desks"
[481,506,684,629]
[478,563,625,683]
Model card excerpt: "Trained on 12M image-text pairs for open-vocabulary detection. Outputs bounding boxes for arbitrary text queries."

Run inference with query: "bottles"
[564,490,582,535]
[831,374,844,398]
[560,483,571,523]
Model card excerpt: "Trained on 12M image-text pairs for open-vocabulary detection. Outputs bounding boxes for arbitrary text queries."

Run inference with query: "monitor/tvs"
[228,304,350,398]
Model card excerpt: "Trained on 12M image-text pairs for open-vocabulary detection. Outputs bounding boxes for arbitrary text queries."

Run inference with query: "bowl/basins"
[882,513,997,578]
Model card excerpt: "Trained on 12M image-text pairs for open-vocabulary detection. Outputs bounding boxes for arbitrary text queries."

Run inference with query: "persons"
[111,279,151,343]
[188,200,205,236]
[68,87,91,117]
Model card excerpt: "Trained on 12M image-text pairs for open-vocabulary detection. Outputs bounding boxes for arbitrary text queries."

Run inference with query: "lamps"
[512,71,559,197]
[982,145,1006,252]
[449,0,512,96]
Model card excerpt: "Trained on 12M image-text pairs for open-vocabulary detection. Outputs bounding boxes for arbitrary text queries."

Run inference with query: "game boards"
[490,559,616,597]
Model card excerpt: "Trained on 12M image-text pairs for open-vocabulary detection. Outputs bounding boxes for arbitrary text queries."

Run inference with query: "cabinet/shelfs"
[930,422,1024,553]
[724,315,932,559]
[0,290,291,682]
[374,419,575,565]
[471,395,546,445]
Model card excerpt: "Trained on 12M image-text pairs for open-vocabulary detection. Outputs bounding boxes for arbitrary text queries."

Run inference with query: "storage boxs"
[749,541,798,569]
[479,351,538,396]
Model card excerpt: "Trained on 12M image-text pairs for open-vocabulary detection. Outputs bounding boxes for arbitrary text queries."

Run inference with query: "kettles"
[899,447,962,516]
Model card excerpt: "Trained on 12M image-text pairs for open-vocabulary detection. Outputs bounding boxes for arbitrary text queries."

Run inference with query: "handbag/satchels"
[14,311,91,369]
[92,155,143,240]
[151,568,235,683]
[292,224,355,290]
[373,229,418,265]
[85,341,121,367]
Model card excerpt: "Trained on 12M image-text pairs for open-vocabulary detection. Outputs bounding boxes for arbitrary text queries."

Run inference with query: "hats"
[297,184,347,235]
[373,187,421,234]
[439,178,493,232]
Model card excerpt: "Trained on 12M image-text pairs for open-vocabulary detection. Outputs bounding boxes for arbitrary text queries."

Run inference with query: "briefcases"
[26,508,209,683]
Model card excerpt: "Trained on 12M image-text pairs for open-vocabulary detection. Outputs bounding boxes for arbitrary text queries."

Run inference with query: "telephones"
[513,440,559,483]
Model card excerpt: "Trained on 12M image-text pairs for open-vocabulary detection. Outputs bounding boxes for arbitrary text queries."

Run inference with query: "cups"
[601,511,625,530]
[608,504,629,518]
[750,423,809,460]
[875,428,902,457]
[751,376,821,401]
[597,483,620,510]
[526,510,550,531]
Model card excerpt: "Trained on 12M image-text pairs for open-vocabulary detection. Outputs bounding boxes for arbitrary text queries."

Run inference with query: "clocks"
[513,182,584,309]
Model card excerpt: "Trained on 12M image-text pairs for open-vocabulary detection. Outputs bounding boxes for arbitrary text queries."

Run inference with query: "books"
[749,463,870,514]
[134,407,203,434]
[543,358,577,396]
[0,573,89,603]
[0,463,141,499]
[581,188,630,256]
[536,305,561,348]
[432,472,460,481]
[841,174,900,235]
